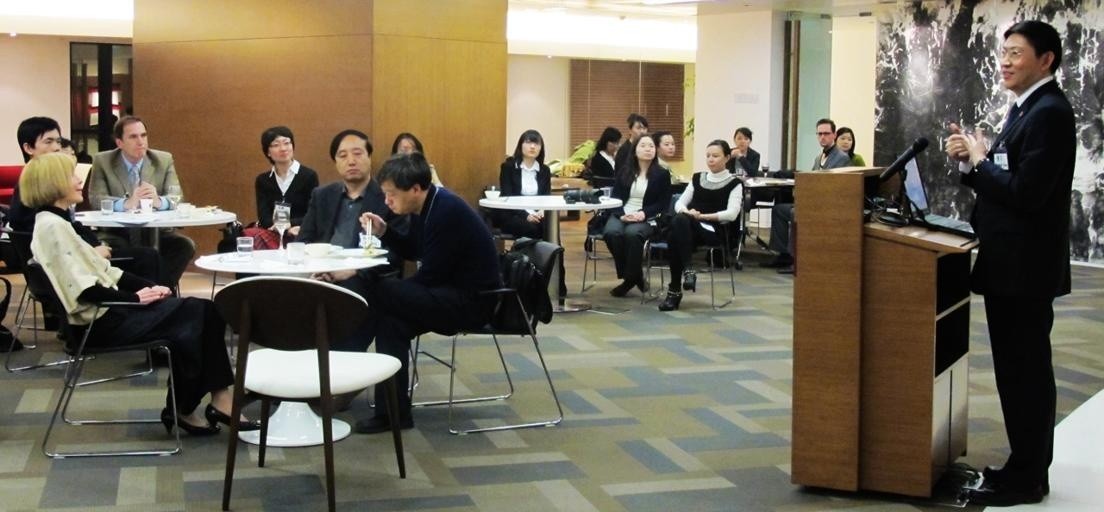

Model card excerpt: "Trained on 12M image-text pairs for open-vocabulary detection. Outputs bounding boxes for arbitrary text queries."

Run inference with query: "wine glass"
[273,211,290,249]
[167,185,182,208]
[762,166,769,177]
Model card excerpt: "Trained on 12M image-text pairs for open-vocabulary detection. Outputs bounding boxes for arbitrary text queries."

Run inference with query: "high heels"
[205,403,263,430]
[683,271,696,292]
[162,408,220,434]
[659,284,682,311]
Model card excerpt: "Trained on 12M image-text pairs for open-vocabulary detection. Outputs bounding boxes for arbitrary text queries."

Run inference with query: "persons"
[497,129,568,305]
[944,19,1077,506]
[761,119,865,268]
[591,114,760,310]
[14,116,197,286]
[20,153,262,436]
[255,127,502,434]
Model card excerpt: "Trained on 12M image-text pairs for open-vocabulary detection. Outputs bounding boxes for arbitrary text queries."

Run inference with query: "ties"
[1006,102,1018,128]
[132,167,141,189]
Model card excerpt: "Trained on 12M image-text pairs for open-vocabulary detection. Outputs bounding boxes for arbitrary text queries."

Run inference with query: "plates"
[342,248,389,258]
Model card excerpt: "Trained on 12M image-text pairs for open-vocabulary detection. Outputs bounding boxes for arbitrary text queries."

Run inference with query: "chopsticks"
[366,217,372,242]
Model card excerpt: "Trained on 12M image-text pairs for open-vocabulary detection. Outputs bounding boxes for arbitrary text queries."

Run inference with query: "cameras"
[564,188,604,204]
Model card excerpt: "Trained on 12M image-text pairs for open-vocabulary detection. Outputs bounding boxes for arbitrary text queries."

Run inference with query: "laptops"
[893,151,978,239]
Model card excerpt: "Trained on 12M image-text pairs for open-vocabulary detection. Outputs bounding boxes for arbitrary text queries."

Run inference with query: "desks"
[670,177,795,266]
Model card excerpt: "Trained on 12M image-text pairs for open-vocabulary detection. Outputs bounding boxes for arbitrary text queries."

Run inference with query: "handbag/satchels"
[491,250,553,332]
[223,221,243,247]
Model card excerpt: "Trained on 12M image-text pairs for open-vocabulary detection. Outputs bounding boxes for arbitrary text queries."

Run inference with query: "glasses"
[999,48,1024,59]
[816,131,831,135]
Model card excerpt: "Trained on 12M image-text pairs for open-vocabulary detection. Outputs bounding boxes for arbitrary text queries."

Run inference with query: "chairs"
[192,246,389,446]
[74,208,238,247]
[479,193,623,312]
[22,256,183,459]
[0,228,154,388]
[642,194,738,311]
[582,188,666,303]
[214,276,406,512]
[410,237,565,435]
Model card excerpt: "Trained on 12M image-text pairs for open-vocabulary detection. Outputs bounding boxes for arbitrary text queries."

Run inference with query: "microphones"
[869,137,928,192]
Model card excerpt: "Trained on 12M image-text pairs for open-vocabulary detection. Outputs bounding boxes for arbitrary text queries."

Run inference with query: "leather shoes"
[969,487,1042,506]
[760,256,793,267]
[357,414,413,432]
[609,276,649,296]
[778,264,793,272]
[984,464,1009,484]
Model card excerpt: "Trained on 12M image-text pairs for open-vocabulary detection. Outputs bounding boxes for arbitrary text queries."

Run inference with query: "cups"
[236,237,254,255]
[141,199,153,215]
[484,190,501,200]
[101,199,114,215]
